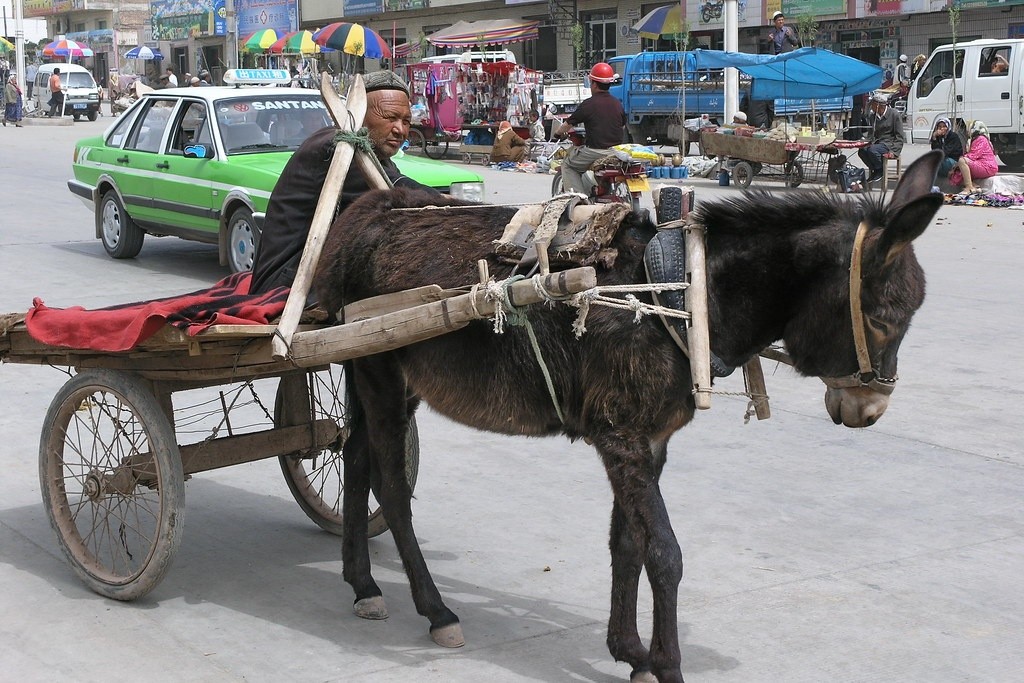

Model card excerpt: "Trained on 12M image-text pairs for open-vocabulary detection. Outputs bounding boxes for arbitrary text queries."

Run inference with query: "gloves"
[912,61,919,73]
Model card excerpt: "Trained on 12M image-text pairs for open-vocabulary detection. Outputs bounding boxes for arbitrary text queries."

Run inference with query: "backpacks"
[828,154,851,184]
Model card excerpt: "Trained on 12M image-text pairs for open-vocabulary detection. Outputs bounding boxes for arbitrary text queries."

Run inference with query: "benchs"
[141,121,264,149]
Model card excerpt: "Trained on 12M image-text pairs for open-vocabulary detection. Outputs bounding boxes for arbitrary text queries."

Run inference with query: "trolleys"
[697,126,876,193]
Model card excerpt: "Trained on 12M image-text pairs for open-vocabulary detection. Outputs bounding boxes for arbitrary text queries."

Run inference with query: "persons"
[97,84,104,117]
[927,116,963,177]
[1,72,24,127]
[108,73,127,116]
[990,53,1009,73]
[490,120,532,164]
[555,62,627,194]
[738,85,774,130]
[528,109,546,142]
[948,120,998,195]
[911,54,927,83]
[247,69,443,296]
[291,110,326,144]
[892,54,911,85]
[46,68,68,118]
[764,10,799,55]
[26,62,38,99]
[159,67,213,112]
[857,94,904,183]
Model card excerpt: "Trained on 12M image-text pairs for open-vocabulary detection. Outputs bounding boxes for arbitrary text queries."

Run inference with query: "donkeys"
[312,150,950,683]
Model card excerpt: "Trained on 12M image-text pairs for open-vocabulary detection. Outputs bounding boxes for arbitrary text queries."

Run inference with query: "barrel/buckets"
[719,170,729,186]
[645,165,688,179]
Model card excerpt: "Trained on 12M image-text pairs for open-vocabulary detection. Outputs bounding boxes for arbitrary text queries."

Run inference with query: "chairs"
[270,119,302,145]
[195,124,229,153]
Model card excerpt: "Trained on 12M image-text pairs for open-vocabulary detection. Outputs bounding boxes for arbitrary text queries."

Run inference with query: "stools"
[869,155,901,193]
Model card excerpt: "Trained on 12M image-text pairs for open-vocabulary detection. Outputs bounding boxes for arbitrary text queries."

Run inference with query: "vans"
[32,63,101,121]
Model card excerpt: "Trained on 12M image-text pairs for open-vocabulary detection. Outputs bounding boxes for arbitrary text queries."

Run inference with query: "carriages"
[0,71,945,683]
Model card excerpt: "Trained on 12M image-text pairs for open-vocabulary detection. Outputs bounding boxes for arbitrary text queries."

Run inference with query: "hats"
[873,95,887,106]
[159,74,168,80]
[191,77,200,83]
[900,54,907,61]
[773,11,783,19]
[362,69,409,99]
[913,54,926,61]
[734,111,748,121]
[202,69,207,73]
[200,71,208,77]
[186,73,191,77]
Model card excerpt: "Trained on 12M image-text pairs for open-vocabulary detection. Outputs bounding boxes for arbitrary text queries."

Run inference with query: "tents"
[688,46,886,188]
[395,19,542,58]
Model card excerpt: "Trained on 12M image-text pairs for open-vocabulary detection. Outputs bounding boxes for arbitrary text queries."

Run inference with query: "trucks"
[601,50,854,148]
[410,49,591,124]
[898,38,1024,167]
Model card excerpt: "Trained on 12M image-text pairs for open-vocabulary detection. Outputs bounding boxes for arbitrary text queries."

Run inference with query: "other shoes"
[866,168,882,183]
[16,124,23,127]
[958,188,976,194]
[3,120,6,126]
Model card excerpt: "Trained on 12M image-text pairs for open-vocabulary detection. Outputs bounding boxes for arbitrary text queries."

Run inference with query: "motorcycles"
[547,103,652,214]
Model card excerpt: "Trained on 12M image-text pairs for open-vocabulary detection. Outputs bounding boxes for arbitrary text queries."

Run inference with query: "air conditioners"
[57,17,68,35]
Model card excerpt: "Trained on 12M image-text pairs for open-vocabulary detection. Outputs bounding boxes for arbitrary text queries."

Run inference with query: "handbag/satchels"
[835,164,867,193]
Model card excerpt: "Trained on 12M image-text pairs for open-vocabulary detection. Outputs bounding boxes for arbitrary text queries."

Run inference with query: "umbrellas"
[629,2,689,40]
[36,38,98,117]
[122,45,164,77]
[238,20,392,76]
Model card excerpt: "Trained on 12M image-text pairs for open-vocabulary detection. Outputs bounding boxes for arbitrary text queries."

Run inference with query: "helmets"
[587,62,614,84]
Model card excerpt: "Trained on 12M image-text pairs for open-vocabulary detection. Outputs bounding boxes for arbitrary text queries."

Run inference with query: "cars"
[68,70,485,276]
